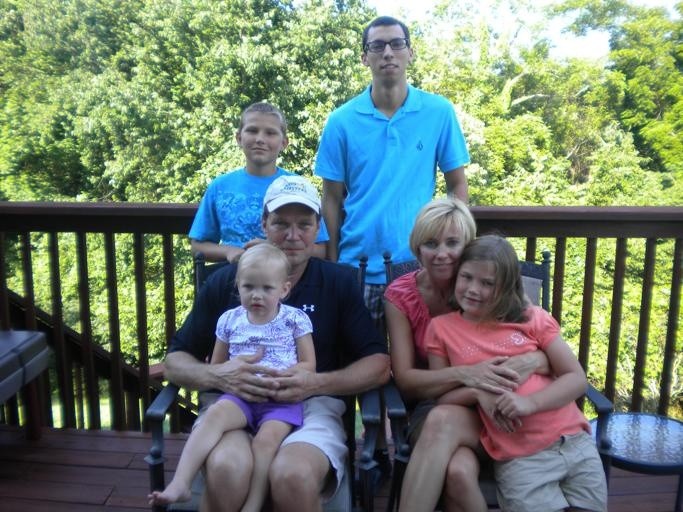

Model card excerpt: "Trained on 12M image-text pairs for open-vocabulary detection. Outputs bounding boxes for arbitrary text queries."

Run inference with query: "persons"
[163,173,390,512]
[146,242,317,512]
[189,104,330,267]
[425,236,608,512]
[311,17,470,480]
[382,197,557,512]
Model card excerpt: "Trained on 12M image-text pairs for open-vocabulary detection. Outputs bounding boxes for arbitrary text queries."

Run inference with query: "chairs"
[144,246,613,511]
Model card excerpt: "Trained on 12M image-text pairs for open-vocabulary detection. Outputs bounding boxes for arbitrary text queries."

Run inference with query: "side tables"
[585,411,682,511]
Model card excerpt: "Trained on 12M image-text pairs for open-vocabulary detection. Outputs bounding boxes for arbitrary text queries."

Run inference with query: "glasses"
[364,38,408,53]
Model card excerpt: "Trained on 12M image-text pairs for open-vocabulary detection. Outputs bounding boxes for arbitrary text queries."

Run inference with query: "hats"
[263,175,323,216]
